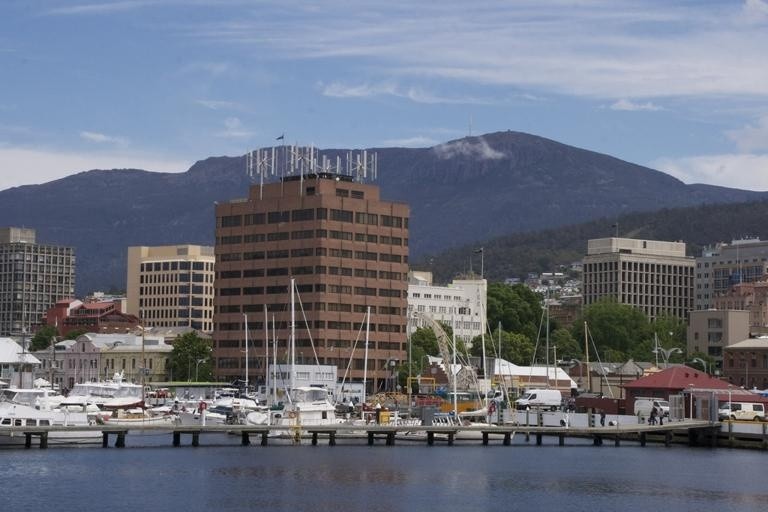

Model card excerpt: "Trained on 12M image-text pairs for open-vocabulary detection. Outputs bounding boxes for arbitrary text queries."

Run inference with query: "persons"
[650,407,656,424]
[569,394,576,412]
[658,409,665,424]
[600,408,605,426]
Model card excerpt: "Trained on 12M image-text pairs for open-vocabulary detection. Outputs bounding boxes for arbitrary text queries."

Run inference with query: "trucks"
[513,388,563,414]
[632,396,670,417]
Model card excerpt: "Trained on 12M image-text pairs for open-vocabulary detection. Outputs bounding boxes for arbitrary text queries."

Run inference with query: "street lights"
[540,304,550,386]
[687,384,694,419]
[552,358,564,390]
[473,245,486,278]
[651,346,683,368]
[726,382,745,441]
[569,358,583,381]
[692,357,706,373]
[668,331,673,347]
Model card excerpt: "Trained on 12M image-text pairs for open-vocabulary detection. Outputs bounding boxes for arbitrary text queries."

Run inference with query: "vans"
[716,401,765,422]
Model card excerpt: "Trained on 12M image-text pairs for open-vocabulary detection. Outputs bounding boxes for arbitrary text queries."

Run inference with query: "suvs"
[563,401,575,413]
